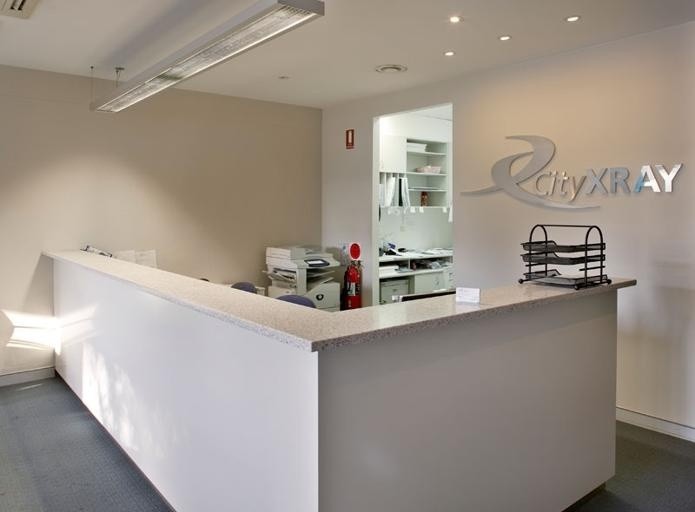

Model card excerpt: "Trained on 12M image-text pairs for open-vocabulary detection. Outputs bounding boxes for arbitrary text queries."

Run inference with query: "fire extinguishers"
[344,259,361,309]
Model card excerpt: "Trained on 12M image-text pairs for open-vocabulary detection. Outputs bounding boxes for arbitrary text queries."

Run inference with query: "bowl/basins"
[418,166,441,174]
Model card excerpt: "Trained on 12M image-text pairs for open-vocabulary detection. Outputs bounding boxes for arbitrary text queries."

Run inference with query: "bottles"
[421,192,428,206]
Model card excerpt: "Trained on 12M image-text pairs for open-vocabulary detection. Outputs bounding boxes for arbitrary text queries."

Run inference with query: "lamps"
[89,0,325,114]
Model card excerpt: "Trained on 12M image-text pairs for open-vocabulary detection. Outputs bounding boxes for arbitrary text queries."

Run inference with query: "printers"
[261,244,342,312]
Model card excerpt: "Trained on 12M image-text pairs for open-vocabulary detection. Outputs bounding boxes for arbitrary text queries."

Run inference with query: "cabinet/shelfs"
[378,128,449,210]
[379,249,455,303]
